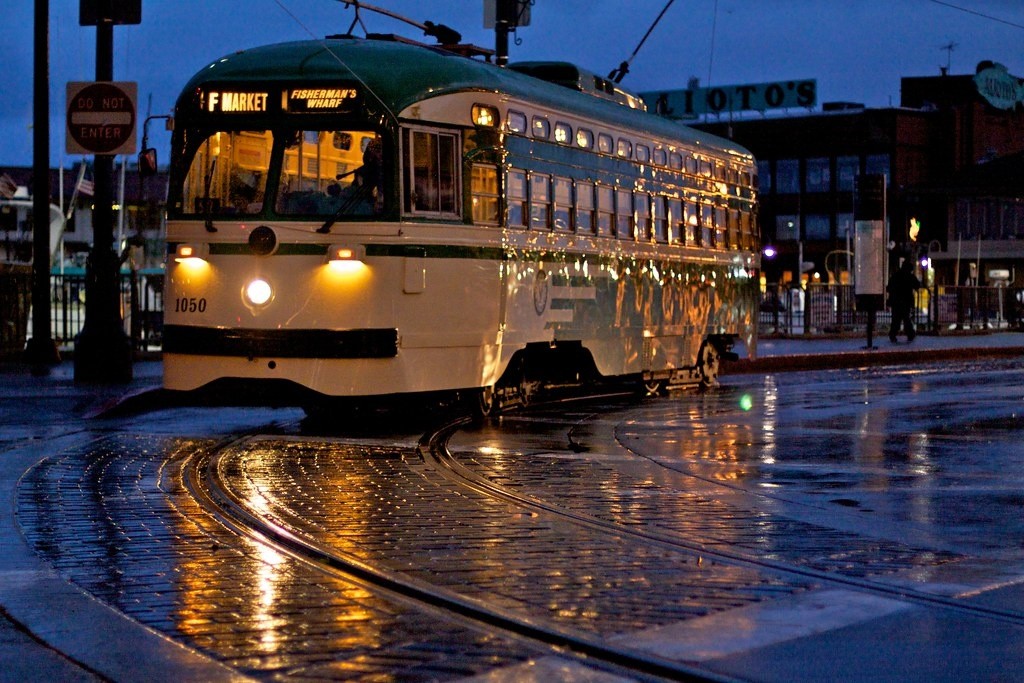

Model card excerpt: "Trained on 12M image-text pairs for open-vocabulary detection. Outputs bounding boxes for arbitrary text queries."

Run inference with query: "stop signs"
[69,84,134,154]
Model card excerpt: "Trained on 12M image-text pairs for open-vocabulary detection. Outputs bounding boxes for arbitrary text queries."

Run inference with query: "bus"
[158,35,771,422]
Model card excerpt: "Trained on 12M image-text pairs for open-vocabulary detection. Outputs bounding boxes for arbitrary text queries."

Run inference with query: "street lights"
[134,115,173,357]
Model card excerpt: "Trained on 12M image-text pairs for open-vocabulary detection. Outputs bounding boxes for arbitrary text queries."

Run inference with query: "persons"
[325,139,384,213]
[888,259,921,342]
